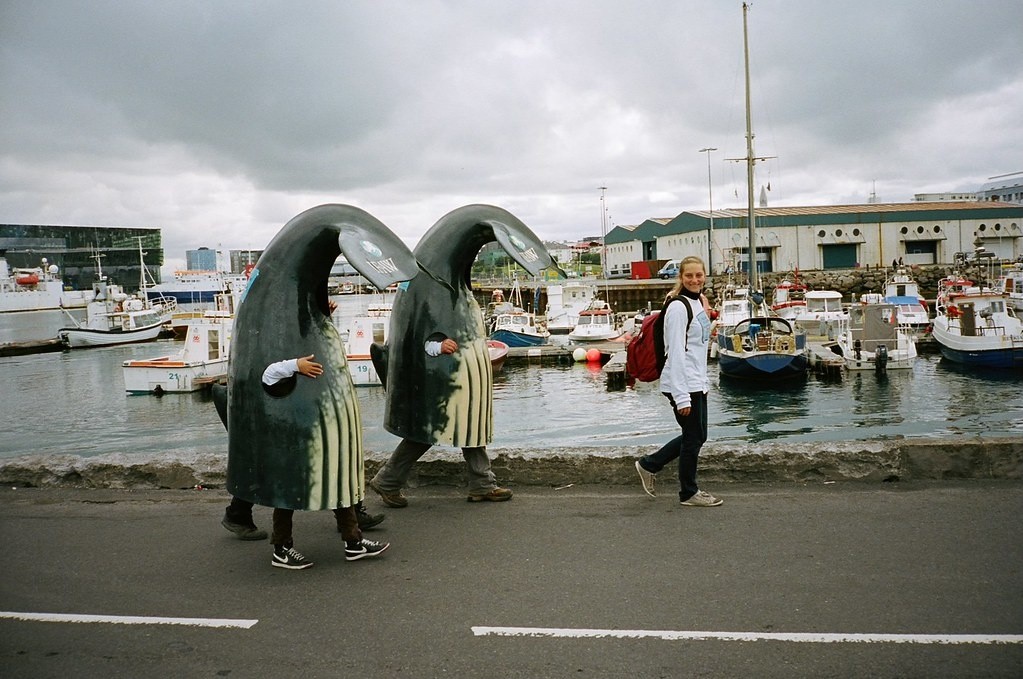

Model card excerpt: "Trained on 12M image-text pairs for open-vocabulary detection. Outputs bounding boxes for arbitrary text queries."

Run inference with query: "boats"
[120,251,635,399]
[57,227,178,351]
[0,256,95,313]
[713,246,1023,373]
[633,301,662,329]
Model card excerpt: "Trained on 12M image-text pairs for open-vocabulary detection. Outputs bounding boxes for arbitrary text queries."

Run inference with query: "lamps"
[979,307,993,321]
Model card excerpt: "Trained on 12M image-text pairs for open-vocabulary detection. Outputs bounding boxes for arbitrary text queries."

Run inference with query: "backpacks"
[625,296,693,382]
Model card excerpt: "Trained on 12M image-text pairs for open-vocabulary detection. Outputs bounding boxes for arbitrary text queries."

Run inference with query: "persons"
[369,331,513,510]
[221,300,392,569]
[635,255,723,507]
[892,257,904,270]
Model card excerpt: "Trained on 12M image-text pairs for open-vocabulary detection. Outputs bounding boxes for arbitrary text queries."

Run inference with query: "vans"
[657,259,682,280]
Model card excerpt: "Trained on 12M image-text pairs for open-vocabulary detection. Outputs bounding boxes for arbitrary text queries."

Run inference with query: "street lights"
[597,187,608,271]
[699,147,718,277]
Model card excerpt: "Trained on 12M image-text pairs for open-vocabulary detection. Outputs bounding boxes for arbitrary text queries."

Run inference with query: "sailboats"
[716,1,808,381]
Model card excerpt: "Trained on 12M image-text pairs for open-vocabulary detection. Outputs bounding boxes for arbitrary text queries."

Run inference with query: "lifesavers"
[776,337,795,354]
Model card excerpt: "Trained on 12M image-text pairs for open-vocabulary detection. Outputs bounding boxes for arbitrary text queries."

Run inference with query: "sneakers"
[272,546,313,570]
[345,538,390,560]
[357,505,386,530]
[221,512,268,540]
[635,461,657,497]
[467,487,513,502]
[370,479,409,508]
[680,490,723,506]
